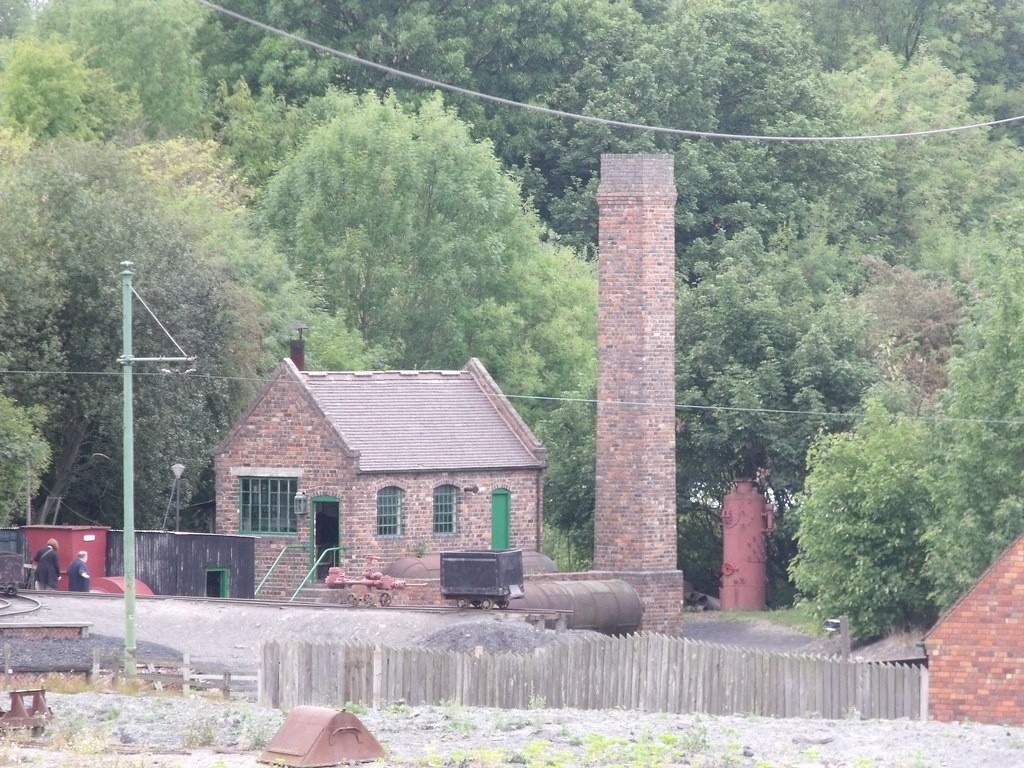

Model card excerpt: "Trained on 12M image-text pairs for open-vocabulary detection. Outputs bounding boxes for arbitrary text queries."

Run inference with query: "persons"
[67,551,91,592]
[33,538,61,591]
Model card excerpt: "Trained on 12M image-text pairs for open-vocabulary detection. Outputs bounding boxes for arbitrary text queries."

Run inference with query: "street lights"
[171,463,184,531]
